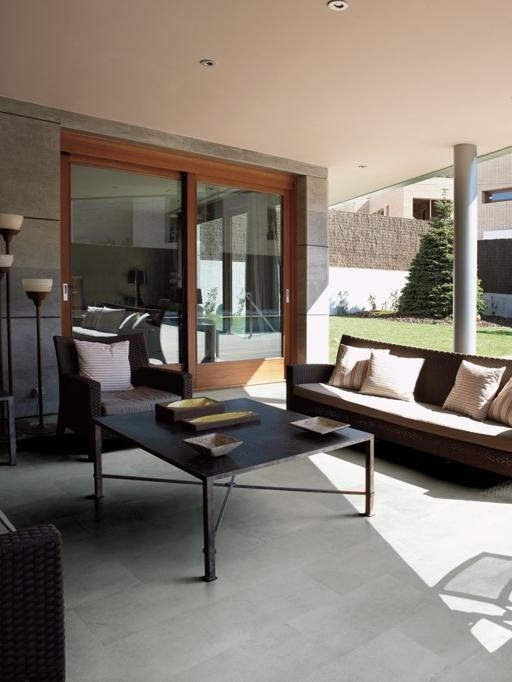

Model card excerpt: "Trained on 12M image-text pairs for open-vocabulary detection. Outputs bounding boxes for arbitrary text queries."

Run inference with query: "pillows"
[75,340,136,391]
[327,342,391,392]
[354,348,427,406]
[443,358,506,422]
[487,373,512,432]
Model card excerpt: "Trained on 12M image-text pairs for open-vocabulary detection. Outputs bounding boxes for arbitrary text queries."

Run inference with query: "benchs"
[286,332,512,491]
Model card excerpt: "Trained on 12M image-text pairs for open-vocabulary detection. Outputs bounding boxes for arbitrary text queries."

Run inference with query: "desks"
[87,396,377,582]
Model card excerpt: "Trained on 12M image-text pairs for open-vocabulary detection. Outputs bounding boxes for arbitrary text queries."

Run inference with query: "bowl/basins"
[183,431,243,460]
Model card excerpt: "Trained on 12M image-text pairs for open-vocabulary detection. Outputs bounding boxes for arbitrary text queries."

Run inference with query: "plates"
[291,413,352,436]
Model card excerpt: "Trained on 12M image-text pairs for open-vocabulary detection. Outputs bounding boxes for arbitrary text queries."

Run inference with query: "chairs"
[47,325,192,462]
[0,508,65,682]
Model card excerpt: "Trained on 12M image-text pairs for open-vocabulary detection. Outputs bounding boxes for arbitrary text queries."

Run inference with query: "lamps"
[0,212,27,396]
[21,277,52,440]
[0,252,13,395]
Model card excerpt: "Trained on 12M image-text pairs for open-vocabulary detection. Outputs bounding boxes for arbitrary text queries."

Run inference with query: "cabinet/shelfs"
[70,195,178,251]
